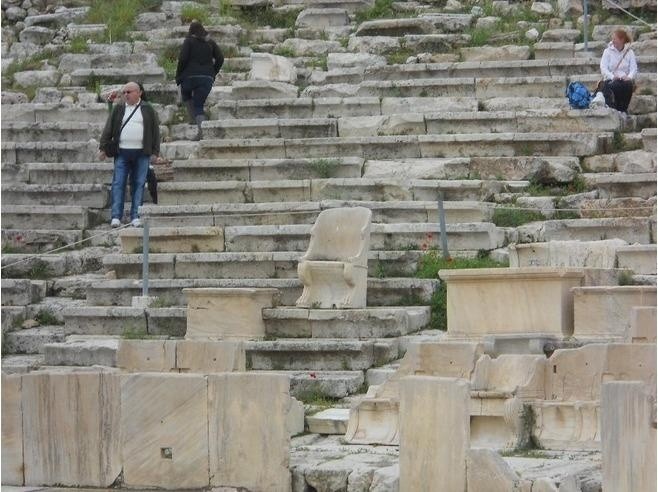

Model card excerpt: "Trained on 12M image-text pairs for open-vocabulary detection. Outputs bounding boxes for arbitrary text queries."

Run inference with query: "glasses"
[122,90,136,94]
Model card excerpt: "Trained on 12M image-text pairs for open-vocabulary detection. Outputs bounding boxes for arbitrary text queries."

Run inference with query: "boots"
[195,114,207,134]
[186,99,197,124]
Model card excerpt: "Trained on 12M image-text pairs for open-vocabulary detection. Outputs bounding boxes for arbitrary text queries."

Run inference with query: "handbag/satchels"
[597,80,607,94]
[104,140,116,158]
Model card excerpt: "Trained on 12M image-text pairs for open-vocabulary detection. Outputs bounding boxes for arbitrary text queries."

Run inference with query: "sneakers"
[111,218,121,228]
[130,218,143,226]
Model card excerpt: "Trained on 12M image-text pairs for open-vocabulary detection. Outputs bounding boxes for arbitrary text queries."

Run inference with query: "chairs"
[296,207,373,308]
[466,351,547,451]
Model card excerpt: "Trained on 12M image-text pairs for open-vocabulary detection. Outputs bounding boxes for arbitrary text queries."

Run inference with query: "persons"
[175,21,224,124]
[598,28,637,113]
[98,81,160,228]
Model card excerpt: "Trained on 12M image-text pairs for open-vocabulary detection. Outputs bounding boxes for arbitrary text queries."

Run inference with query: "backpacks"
[564,80,594,109]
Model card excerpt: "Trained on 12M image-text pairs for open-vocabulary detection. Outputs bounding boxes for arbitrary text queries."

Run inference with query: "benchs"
[341,343,488,447]
[513,342,656,448]
